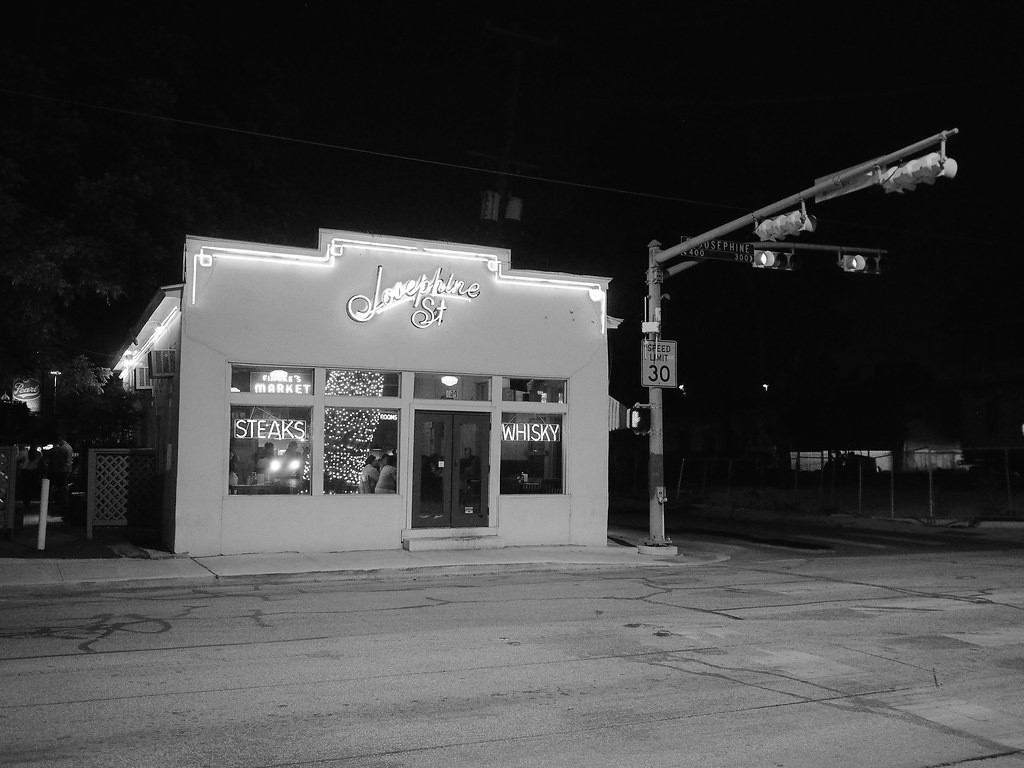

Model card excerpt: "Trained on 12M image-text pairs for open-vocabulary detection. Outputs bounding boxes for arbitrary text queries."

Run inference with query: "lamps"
[441,375,458,387]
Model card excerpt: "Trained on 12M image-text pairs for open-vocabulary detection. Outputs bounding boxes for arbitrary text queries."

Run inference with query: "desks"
[231,485,269,493]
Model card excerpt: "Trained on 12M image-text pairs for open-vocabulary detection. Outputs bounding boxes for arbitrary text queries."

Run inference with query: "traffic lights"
[755,211,816,242]
[752,250,800,271]
[881,153,958,192]
[839,255,880,275]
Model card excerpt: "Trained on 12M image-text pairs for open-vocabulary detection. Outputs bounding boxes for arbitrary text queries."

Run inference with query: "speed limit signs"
[640,339,677,388]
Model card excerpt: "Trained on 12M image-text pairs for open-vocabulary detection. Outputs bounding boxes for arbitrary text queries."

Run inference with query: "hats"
[366,455,376,464]
[382,454,390,459]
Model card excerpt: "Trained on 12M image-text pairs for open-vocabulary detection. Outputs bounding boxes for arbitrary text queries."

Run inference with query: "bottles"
[251,472,257,484]
[247,476,251,484]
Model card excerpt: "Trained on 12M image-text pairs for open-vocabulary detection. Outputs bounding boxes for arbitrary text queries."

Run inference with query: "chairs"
[500,477,562,494]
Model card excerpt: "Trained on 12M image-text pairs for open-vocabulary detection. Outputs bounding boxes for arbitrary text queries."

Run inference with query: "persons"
[464,448,472,459]
[362,455,380,481]
[375,456,397,493]
[51,434,73,505]
[260,442,275,458]
[18,441,42,510]
[229,449,240,494]
[284,442,298,457]
